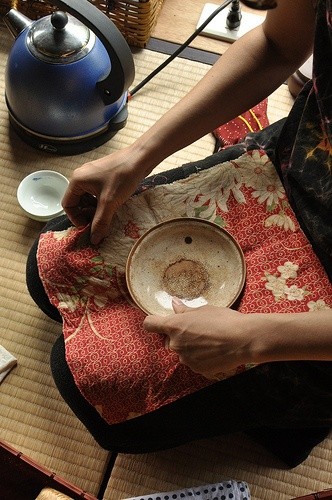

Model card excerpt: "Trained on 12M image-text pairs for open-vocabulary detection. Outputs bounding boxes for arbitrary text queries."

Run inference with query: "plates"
[125,217,247,315]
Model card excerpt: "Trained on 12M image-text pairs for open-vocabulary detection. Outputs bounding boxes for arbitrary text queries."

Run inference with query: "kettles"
[2,0,136,143]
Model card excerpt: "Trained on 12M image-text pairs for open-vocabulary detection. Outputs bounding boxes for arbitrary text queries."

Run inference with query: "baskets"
[1,0,167,48]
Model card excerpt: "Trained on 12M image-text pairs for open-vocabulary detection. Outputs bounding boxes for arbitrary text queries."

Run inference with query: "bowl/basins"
[17,169,70,222]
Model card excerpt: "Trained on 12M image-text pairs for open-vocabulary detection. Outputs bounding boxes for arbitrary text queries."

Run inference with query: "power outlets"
[197,3,265,44]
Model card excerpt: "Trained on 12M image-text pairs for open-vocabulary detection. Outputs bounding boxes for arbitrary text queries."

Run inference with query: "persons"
[25,0,332,467]
